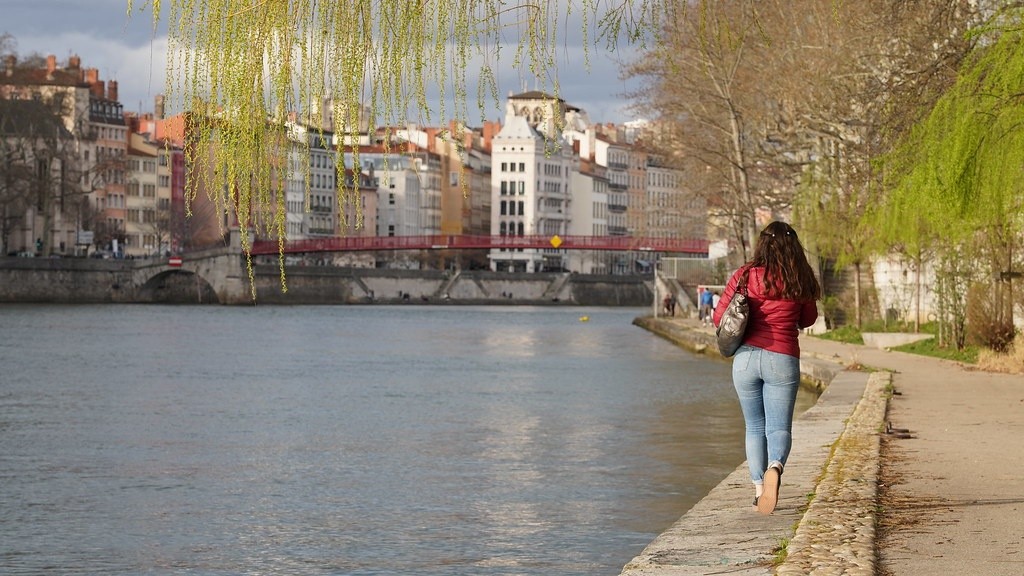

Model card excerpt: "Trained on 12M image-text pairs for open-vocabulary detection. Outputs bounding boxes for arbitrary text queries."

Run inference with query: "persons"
[700,287,721,327]
[663,294,676,317]
[714,221,821,514]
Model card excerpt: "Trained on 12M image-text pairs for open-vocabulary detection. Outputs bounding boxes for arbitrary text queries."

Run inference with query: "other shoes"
[709,323,713,327]
[758,466,781,515]
[703,324,706,327]
[752,497,759,512]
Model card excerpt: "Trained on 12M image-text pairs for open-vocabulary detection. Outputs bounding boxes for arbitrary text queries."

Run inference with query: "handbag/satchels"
[716,262,751,358]
